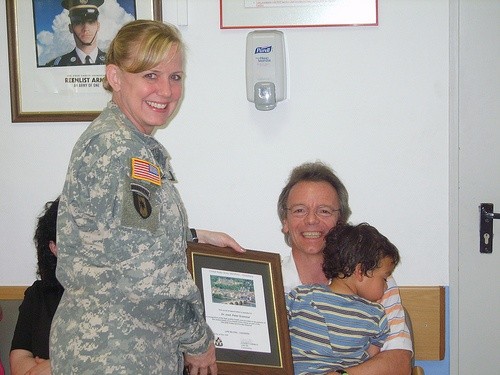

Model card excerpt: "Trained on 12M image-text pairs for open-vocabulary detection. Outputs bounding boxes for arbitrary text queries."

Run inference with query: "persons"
[9,196,65,375]
[46,0,107,67]
[48,19,246,375]
[284,220,401,375]
[277,161,414,375]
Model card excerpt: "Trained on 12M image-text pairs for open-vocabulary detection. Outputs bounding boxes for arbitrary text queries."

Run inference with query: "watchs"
[190,228,198,243]
[336,369,349,375]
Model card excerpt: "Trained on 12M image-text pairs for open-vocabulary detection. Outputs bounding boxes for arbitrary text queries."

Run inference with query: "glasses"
[284,205,341,217]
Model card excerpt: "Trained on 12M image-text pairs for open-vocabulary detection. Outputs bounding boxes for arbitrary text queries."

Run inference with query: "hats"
[62,0,104,21]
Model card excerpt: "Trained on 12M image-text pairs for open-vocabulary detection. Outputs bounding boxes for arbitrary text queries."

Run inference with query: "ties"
[85,56,91,65]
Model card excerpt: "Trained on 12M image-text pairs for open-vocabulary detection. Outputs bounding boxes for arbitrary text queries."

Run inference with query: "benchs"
[0,286,446,375]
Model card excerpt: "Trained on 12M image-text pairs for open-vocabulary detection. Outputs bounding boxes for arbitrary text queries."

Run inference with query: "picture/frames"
[180,243,294,375]
[6,0,163,123]
